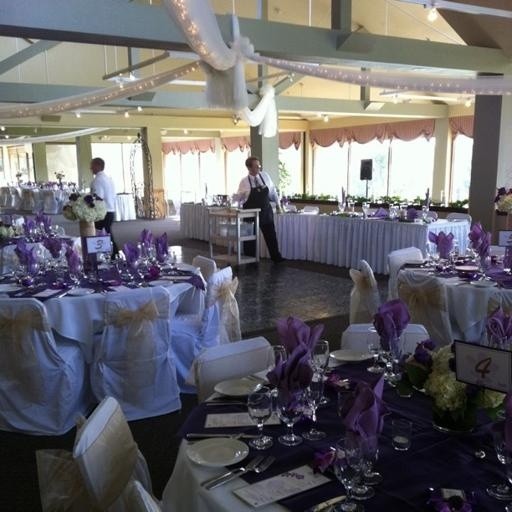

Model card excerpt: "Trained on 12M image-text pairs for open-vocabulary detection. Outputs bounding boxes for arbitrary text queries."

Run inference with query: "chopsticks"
[41,287,72,303]
[9,285,46,297]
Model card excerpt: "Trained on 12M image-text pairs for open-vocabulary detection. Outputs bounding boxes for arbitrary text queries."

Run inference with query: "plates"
[67,288,95,296]
[455,265,479,272]
[432,420,494,436]
[404,260,424,264]
[214,379,261,396]
[173,264,194,272]
[470,280,498,287]
[329,349,373,362]
[187,438,249,467]
[149,280,173,286]
[367,217,380,220]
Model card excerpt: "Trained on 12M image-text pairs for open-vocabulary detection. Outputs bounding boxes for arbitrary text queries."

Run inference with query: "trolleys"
[200,196,262,268]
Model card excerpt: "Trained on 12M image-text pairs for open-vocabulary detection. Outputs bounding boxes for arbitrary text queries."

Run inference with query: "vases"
[59,182,63,190]
[79,224,93,270]
[79,221,97,275]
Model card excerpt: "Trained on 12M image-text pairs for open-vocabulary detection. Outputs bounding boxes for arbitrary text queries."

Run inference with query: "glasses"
[256,165,262,168]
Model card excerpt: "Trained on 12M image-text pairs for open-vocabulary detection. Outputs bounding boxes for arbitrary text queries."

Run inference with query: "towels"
[469,221,482,248]
[458,273,482,281]
[429,231,454,259]
[475,231,491,260]
[102,267,122,286]
[65,248,81,267]
[122,240,140,268]
[486,305,512,343]
[485,266,512,281]
[14,239,36,265]
[280,196,288,203]
[186,275,205,291]
[155,232,168,261]
[22,218,34,228]
[313,371,392,475]
[407,208,417,219]
[372,207,389,217]
[35,212,51,226]
[266,342,314,411]
[141,228,153,248]
[277,316,324,353]
[503,250,512,268]
[43,238,62,258]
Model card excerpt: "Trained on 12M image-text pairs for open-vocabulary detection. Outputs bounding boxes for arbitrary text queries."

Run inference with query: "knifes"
[188,434,267,439]
[307,495,347,511]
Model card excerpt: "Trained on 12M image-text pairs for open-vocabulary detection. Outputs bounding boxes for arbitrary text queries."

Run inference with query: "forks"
[206,457,276,491]
[205,400,260,406]
[201,456,264,486]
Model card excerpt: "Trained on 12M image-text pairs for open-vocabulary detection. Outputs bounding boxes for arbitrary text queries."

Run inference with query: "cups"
[392,420,412,451]
[338,392,351,416]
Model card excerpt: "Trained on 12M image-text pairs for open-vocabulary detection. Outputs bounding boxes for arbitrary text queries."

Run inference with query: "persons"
[237,157,287,266]
[89,158,119,256]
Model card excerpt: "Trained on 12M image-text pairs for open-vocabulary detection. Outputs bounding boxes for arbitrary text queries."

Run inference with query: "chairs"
[195,336,275,404]
[448,213,474,220]
[192,255,217,282]
[43,192,58,215]
[171,265,238,394]
[1,297,84,437]
[0,186,21,210]
[73,395,162,511]
[349,259,382,329]
[91,286,182,422]
[21,190,33,210]
[343,324,428,354]
[387,247,423,301]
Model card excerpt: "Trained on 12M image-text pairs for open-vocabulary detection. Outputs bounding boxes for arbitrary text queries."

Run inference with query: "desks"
[179,204,472,275]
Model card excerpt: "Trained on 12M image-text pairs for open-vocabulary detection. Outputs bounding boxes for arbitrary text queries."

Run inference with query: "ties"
[254,177,261,187]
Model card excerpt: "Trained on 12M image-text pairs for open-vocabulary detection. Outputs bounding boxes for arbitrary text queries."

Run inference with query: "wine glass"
[489,336,507,351]
[362,202,370,220]
[367,327,384,373]
[333,437,383,512]
[276,388,304,447]
[348,203,355,218]
[126,242,173,286]
[30,224,58,242]
[302,374,326,441]
[487,433,512,500]
[71,259,83,285]
[380,337,404,389]
[247,393,273,450]
[264,346,286,393]
[14,249,68,286]
[311,341,329,404]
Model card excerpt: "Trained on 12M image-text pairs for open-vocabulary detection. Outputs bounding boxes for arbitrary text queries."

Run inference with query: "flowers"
[471,387,501,418]
[412,337,434,359]
[54,172,65,179]
[62,193,107,222]
[422,343,460,414]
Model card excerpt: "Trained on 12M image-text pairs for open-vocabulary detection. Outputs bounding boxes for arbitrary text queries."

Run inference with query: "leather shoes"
[274,258,288,265]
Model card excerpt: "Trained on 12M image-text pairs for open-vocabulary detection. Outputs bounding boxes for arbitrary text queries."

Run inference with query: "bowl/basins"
[220,222,253,238]
